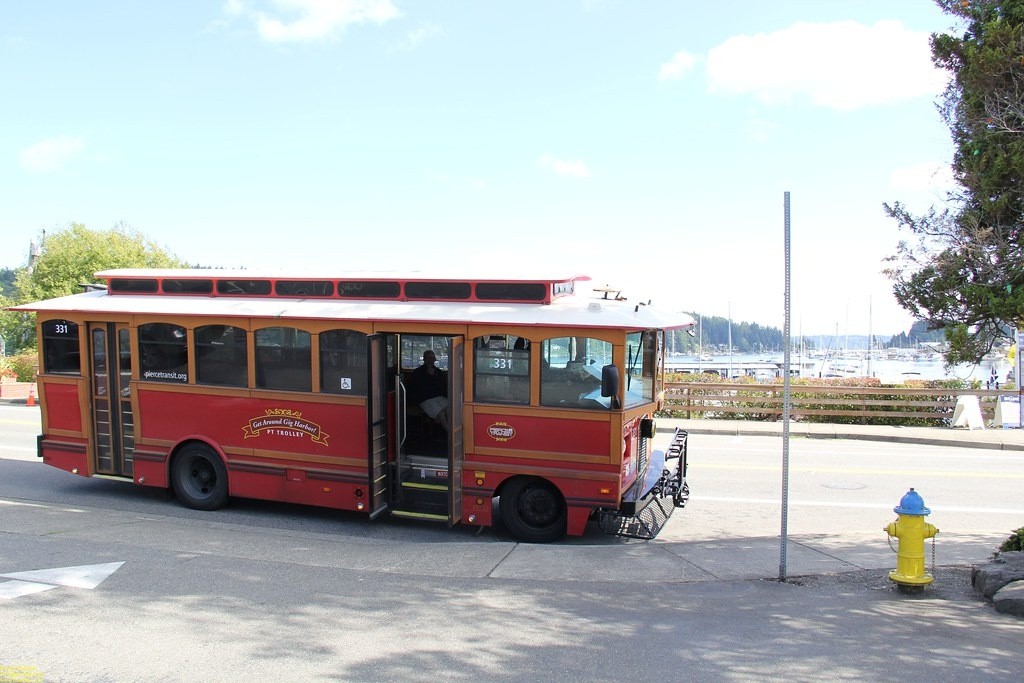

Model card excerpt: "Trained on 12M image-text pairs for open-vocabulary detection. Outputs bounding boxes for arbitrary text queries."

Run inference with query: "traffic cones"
[25,382,37,407]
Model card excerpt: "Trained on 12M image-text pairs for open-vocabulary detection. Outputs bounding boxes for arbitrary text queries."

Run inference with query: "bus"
[6,269,697,542]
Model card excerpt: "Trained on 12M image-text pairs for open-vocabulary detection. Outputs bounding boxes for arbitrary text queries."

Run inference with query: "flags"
[1007,342,1015,366]
[990,365,998,384]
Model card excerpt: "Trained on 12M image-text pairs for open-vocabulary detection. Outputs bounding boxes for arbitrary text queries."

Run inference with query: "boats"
[696,355,713,361]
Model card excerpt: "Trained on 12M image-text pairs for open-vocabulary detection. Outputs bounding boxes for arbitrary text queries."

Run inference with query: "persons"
[409,350,449,434]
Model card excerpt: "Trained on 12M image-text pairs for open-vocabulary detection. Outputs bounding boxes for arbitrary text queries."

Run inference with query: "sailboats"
[760,294,1005,379]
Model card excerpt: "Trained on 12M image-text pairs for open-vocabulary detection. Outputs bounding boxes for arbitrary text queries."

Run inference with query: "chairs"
[512,337,561,406]
[399,385,434,436]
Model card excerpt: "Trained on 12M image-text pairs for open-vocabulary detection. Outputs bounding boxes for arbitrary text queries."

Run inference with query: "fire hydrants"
[884,488,941,591]
